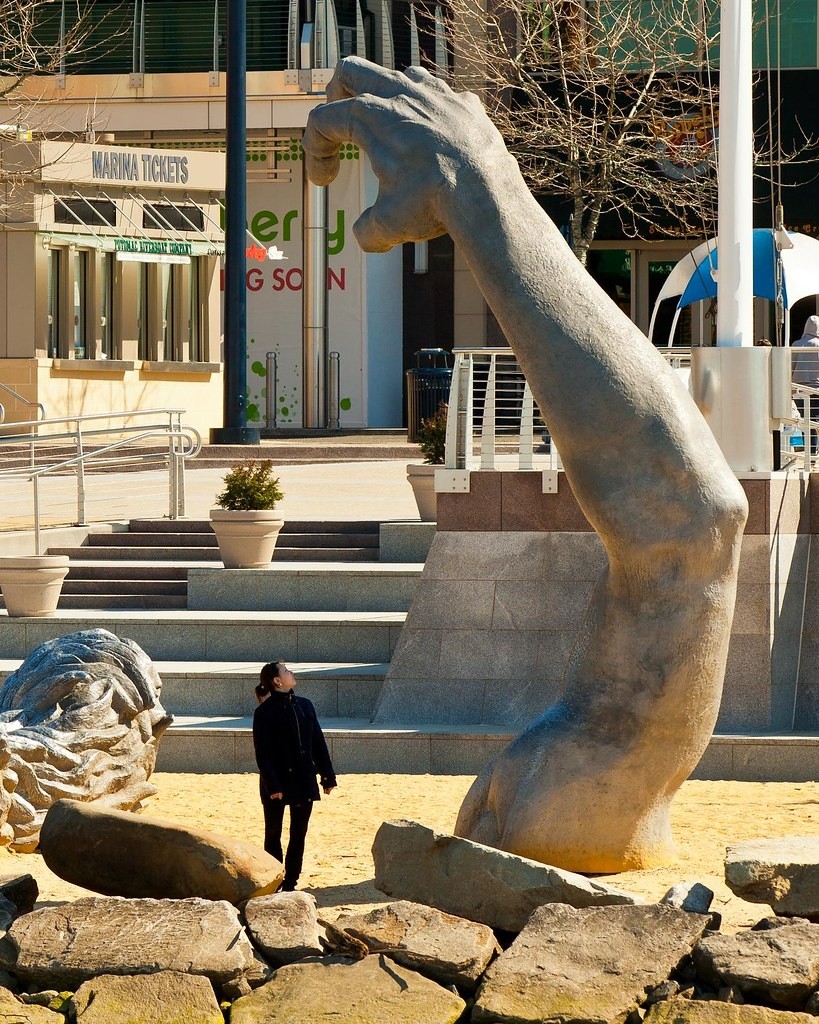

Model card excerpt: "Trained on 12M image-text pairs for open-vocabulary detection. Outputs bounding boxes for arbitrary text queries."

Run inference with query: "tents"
[647,228,819,368]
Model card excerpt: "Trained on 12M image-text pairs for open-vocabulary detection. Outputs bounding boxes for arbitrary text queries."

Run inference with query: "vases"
[0,556,69,617]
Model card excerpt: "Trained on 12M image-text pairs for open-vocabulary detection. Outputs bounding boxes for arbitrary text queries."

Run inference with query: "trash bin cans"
[405,347,453,443]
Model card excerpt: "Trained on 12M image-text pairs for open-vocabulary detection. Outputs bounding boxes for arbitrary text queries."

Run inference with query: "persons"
[252,661,337,892]
[300,57,750,871]
[756,338,772,346]
[790,315,819,466]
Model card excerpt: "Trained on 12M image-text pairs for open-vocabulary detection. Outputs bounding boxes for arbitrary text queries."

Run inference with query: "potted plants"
[209,448,289,572]
[404,399,475,522]
